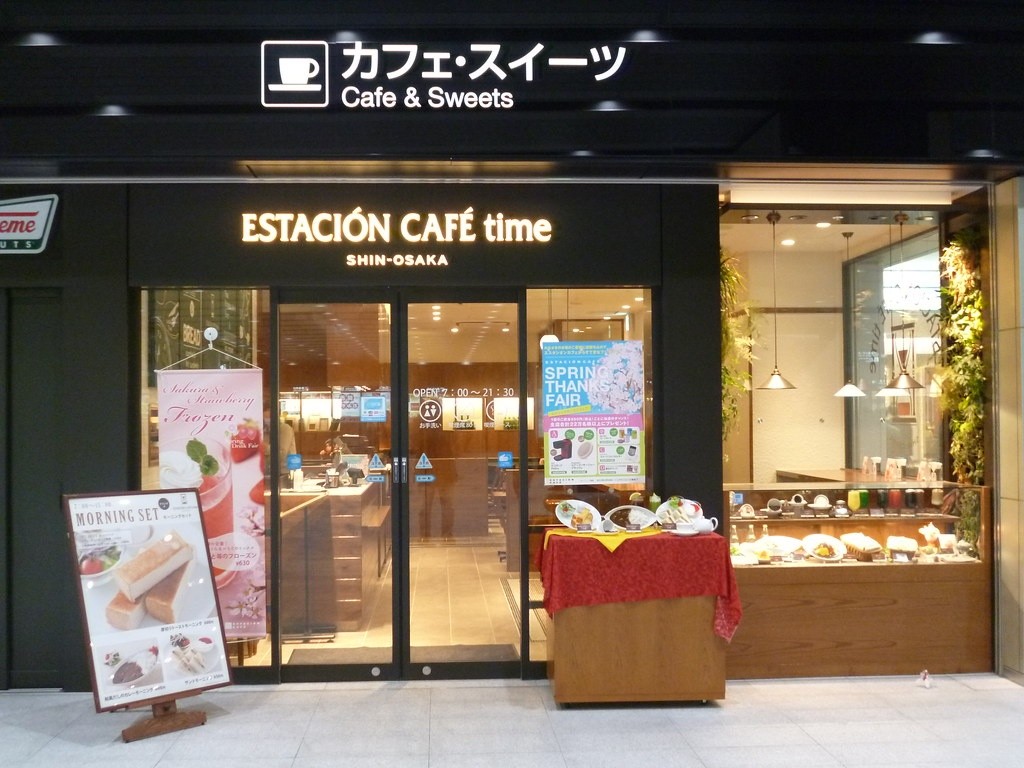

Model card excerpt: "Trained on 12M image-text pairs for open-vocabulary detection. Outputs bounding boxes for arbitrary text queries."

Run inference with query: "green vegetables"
[185,439,217,476]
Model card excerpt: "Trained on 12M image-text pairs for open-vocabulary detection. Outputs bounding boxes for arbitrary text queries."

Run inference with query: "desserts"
[105,530,198,629]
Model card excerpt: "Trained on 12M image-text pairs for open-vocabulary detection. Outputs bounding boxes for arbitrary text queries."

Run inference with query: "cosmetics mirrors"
[767,498,782,511]
[792,493,803,503]
[814,494,829,506]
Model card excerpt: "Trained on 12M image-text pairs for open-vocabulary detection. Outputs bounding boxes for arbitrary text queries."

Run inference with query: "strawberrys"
[228,419,265,504]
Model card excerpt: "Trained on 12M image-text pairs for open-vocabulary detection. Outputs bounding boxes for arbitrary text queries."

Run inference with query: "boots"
[441,504,456,538]
[418,507,432,538]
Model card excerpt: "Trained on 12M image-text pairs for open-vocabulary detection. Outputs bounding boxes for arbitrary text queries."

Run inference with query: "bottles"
[730,525,740,555]
[459,415,466,428]
[503,417,520,429]
[760,524,769,540]
[453,416,459,428]
[745,524,756,544]
[465,415,472,429]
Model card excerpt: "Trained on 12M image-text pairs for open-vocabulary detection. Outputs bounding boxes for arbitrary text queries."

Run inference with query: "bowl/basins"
[79,549,122,586]
[106,649,164,690]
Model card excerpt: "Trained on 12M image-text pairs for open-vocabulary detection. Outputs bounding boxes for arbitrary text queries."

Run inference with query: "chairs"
[487,464,512,532]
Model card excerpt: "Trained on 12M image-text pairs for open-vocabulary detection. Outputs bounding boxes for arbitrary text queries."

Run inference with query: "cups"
[623,434,630,443]
[632,430,637,439]
[871,457,881,472]
[577,524,593,533]
[929,462,942,481]
[619,430,625,438]
[631,495,644,508]
[677,523,693,532]
[159,435,237,589]
[649,496,661,513]
[661,523,676,532]
[319,418,329,431]
[895,458,907,477]
[626,524,641,532]
[627,428,632,436]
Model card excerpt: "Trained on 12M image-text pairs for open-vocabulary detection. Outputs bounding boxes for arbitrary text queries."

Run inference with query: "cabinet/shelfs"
[536,527,743,706]
[724,468,992,679]
[278,464,391,631]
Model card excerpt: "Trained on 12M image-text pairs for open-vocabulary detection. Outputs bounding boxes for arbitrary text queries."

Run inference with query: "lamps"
[831,232,866,397]
[540,289,559,350]
[885,214,924,389]
[756,211,796,390]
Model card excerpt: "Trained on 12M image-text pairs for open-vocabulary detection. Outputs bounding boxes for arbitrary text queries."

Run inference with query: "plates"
[939,554,976,563]
[801,534,847,560]
[604,505,658,533]
[170,645,220,677]
[555,499,601,531]
[672,529,699,536]
[655,499,703,536]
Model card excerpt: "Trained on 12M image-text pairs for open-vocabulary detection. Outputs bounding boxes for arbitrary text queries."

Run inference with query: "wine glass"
[931,489,944,517]
[858,490,870,519]
[869,490,880,519]
[888,489,902,518]
[915,489,925,513]
[847,489,860,519]
[634,466,638,473]
[877,489,888,518]
[905,489,916,518]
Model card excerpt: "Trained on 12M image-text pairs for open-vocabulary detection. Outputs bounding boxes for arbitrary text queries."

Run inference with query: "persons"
[320,439,332,460]
[410,393,460,546]
[263,400,297,475]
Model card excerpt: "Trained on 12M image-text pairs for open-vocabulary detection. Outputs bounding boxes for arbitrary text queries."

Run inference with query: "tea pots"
[689,516,719,535]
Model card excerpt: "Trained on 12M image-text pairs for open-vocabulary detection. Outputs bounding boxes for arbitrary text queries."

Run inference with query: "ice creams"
[158,451,203,494]
